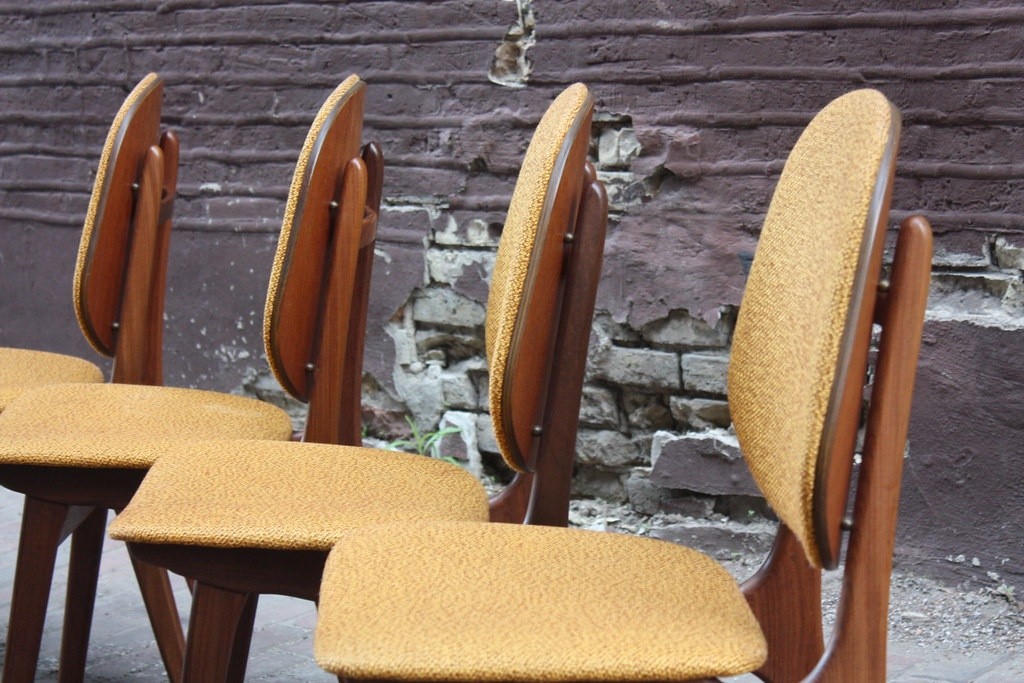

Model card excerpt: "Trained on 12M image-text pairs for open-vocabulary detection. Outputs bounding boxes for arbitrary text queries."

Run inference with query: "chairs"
[0,72,195,683]
[0,70,385,683]
[310,90,937,683]
[114,84,608,683]
